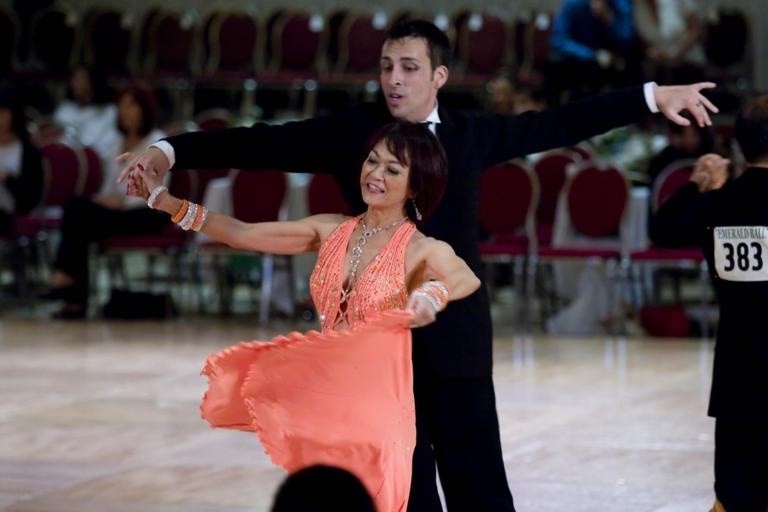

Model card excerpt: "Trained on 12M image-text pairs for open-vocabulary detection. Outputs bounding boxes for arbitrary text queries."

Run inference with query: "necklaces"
[338,211,410,303]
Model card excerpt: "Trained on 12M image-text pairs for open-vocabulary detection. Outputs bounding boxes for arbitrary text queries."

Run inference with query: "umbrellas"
[35,280,84,301]
[51,306,87,320]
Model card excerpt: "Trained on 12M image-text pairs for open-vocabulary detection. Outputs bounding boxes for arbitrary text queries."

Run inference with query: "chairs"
[0,0,767,337]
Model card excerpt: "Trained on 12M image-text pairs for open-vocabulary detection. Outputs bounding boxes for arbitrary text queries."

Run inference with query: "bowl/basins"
[338,211,410,303]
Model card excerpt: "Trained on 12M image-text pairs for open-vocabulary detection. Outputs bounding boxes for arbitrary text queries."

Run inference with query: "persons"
[50,70,123,286]
[124,119,482,512]
[1,100,42,285]
[269,463,377,512]
[118,18,722,512]
[549,0,750,187]
[39,81,172,318]
[647,92,768,512]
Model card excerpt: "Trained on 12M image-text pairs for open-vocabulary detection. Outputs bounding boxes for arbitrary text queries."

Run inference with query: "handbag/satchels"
[147,186,207,236]
[409,280,450,315]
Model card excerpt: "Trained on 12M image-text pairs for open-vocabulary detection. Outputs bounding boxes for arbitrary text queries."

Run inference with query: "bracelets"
[409,280,450,315]
[147,186,207,236]
